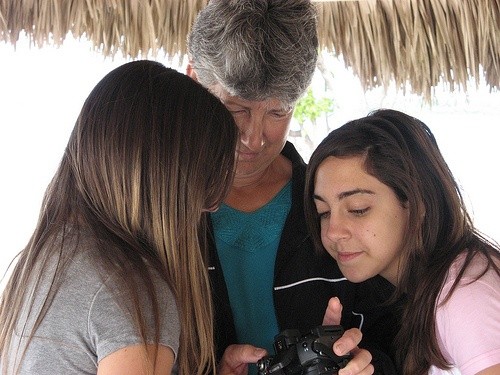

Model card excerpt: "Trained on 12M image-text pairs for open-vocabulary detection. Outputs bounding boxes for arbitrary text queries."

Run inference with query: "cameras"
[257,325,355,375]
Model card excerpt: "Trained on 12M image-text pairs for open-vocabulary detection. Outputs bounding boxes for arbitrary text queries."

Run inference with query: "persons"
[0,59,240,375]
[186,1,377,375]
[306,108,500,375]
